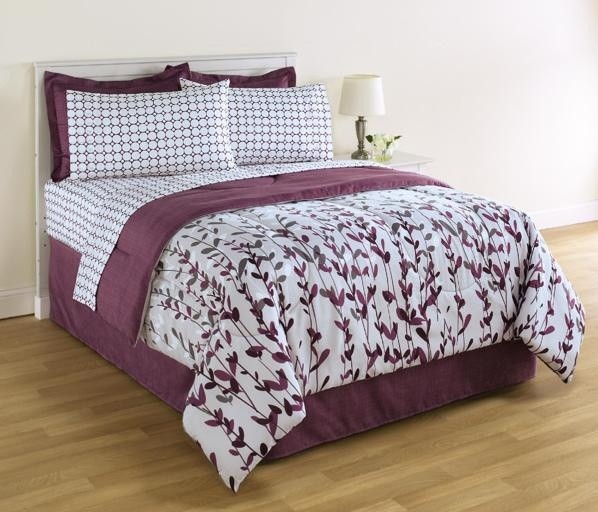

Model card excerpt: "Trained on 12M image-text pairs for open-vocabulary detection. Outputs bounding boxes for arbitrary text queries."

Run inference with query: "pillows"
[42,61,334,183]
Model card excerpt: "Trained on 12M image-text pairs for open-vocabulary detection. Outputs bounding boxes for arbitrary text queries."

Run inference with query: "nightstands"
[390,150,433,173]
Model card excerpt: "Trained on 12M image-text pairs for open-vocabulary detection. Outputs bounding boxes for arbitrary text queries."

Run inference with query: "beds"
[35,52,587,494]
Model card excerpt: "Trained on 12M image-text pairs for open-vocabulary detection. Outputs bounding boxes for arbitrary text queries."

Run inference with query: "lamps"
[339,74,386,161]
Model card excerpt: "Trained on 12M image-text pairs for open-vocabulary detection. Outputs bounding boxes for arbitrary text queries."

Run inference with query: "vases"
[373,149,392,162]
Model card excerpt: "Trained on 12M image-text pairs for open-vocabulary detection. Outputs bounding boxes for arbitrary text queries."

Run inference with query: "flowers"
[366,134,402,149]
